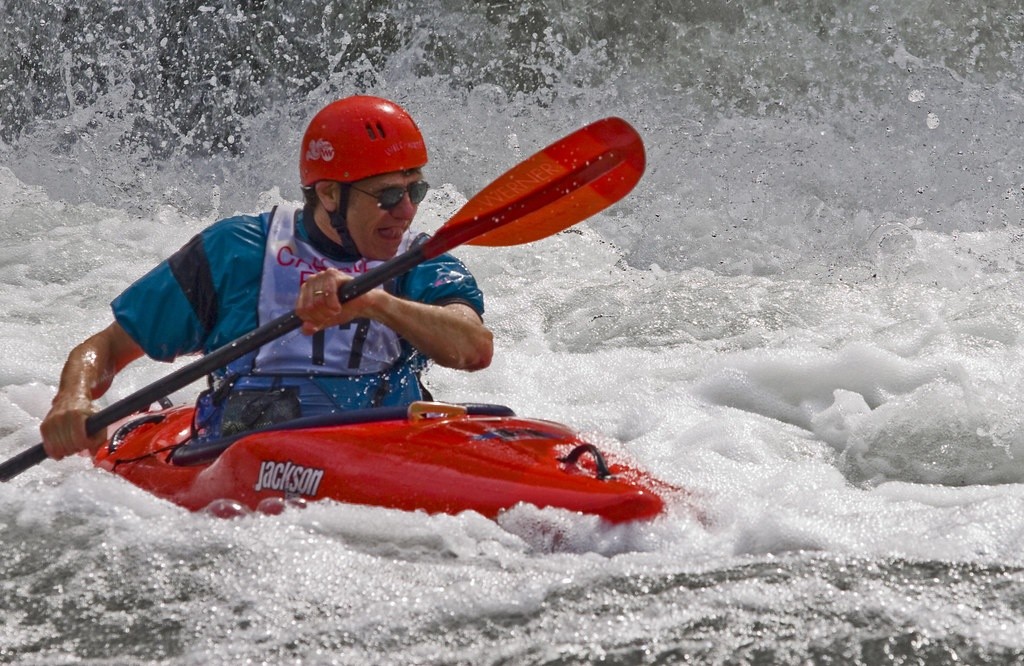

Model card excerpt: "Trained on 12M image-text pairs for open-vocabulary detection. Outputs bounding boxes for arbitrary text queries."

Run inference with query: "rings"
[313,290,324,294]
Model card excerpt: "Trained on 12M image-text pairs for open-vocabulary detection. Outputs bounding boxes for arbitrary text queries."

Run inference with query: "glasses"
[345,181,429,209]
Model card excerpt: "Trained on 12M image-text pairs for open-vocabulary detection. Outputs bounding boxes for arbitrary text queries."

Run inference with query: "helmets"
[300,96,428,186]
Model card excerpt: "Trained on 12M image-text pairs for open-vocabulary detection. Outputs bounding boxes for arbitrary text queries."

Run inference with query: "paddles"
[0,117,645,482]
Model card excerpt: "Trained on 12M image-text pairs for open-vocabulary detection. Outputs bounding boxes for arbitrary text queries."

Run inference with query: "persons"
[37,95,494,467]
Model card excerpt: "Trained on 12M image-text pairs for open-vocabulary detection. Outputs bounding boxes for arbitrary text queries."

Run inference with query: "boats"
[92,397,693,524]
